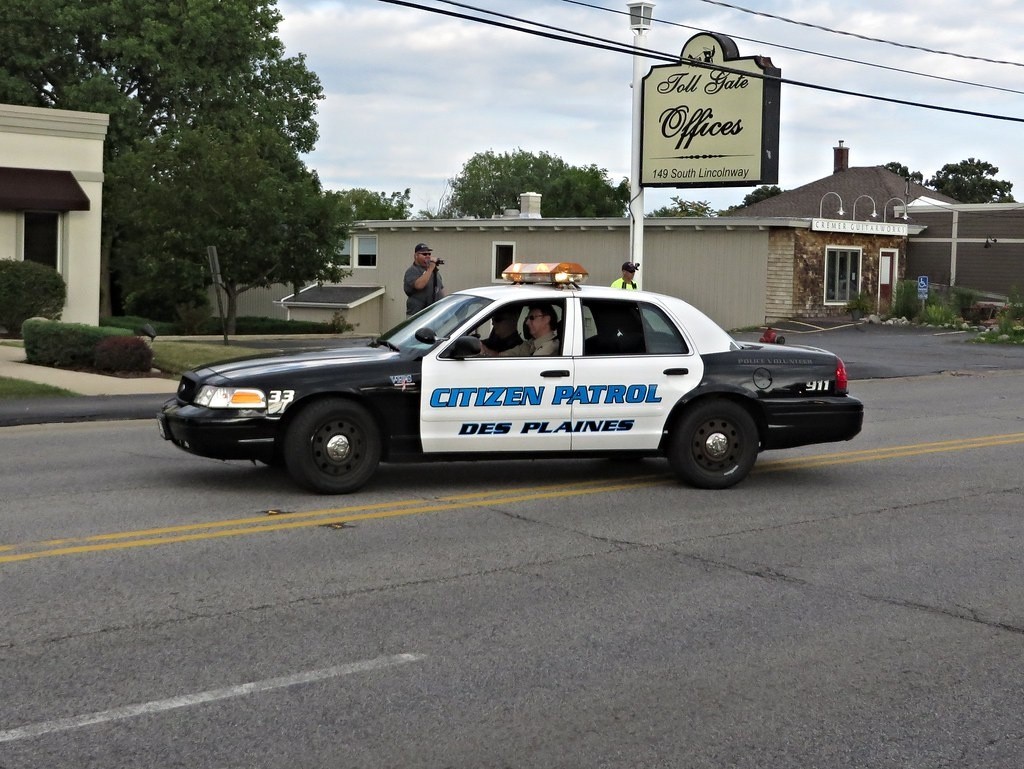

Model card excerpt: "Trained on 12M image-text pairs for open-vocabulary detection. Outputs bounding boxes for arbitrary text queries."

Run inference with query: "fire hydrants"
[761,326,787,346]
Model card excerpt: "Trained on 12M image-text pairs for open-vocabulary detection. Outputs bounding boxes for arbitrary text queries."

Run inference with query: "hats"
[622,262,638,271]
[415,243,432,253]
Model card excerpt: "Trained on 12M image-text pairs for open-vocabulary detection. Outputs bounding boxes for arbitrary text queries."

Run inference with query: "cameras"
[435,258,445,267]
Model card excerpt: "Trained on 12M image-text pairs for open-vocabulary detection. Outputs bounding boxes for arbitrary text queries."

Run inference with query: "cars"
[157,263,865,490]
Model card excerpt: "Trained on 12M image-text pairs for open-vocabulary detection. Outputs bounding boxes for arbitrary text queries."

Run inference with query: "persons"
[469,302,559,356]
[611,262,639,291]
[404,243,447,341]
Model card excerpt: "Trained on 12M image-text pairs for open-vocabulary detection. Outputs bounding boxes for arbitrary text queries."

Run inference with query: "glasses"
[417,253,431,256]
[526,315,545,320]
[627,270,635,273]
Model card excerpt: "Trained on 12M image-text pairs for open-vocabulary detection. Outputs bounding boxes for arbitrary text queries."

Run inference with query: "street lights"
[628,1,656,292]
